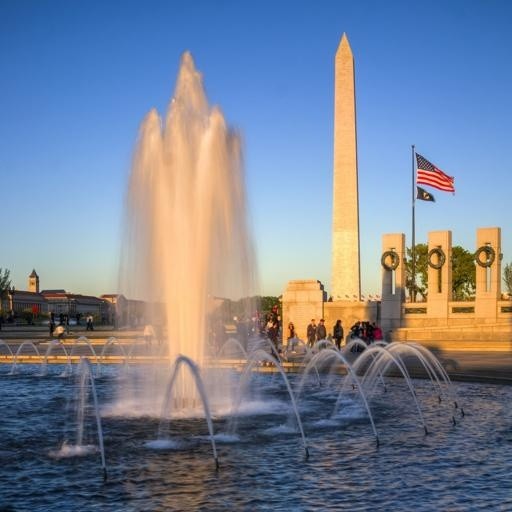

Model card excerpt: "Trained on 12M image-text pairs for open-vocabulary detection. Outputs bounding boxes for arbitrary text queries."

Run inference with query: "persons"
[86,312,94,331]
[212,305,383,353]
[64,312,69,324]
[101,312,105,325]
[49,312,55,335]
[75,311,80,324]
[142,322,154,353]
[59,312,66,324]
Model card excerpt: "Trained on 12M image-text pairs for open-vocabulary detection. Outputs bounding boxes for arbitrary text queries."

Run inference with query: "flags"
[416,152,456,193]
[416,186,434,201]
